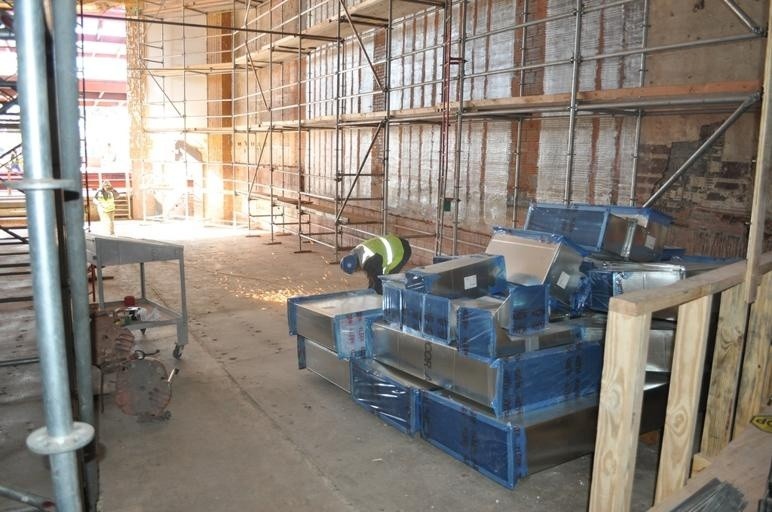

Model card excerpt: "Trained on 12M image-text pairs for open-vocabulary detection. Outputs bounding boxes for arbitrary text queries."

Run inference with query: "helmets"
[340,255,356,273]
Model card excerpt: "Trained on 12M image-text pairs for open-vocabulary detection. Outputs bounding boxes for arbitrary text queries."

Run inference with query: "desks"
[85,232,189,358]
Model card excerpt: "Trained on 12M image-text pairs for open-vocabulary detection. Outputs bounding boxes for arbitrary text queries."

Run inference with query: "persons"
[92,179,120,234]
[340,234,411,293]
[9,149,21,172]
